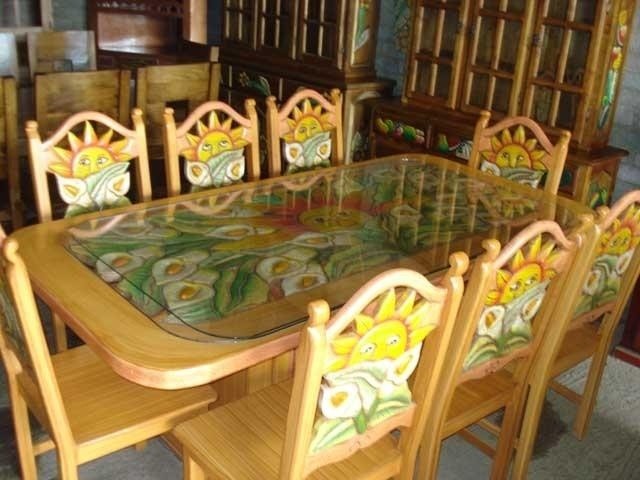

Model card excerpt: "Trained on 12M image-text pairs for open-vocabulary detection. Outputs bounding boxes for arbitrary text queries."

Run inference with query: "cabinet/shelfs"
[218,66,343,147]
[401,1,637,153]
[221,1,379,80]
[366,114,480,170]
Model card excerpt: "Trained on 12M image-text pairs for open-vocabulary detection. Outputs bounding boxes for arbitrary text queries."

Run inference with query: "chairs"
[468,191,640,478]
[1,73,24,231]
[27,30,97,84]
[24,105,153,354]
[30,69,131,212]
[1,31,20,88]
[132,62,222,174]
[175,38,218,66]
[471,108,570,195]
[267,90,345,175]
[1,227,223,479]
[174,247,464,477]
[161,97,260,200]
[397,218,585,479]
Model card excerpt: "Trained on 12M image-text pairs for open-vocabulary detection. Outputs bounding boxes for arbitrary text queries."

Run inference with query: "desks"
[9,154,612,462]
